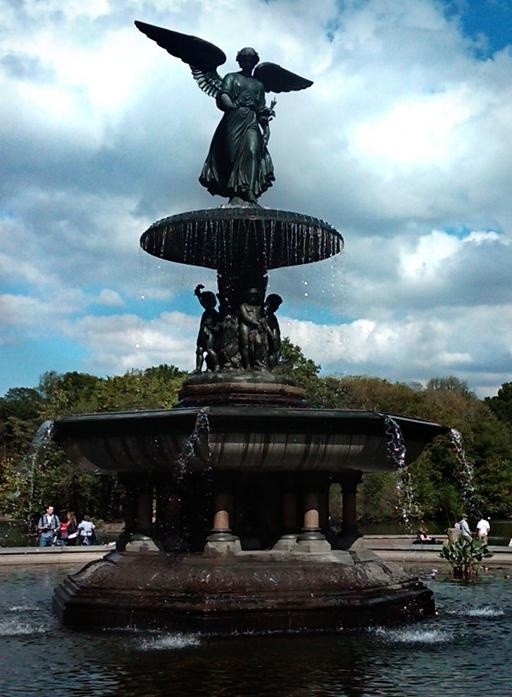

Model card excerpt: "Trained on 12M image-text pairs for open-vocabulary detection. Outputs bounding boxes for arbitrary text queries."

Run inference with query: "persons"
[476,515,491,548]
[412,524,439,544]
[188,284,282,376]
[135,21,313,211]
[37,505,97,547]
[456,512,473,547]
